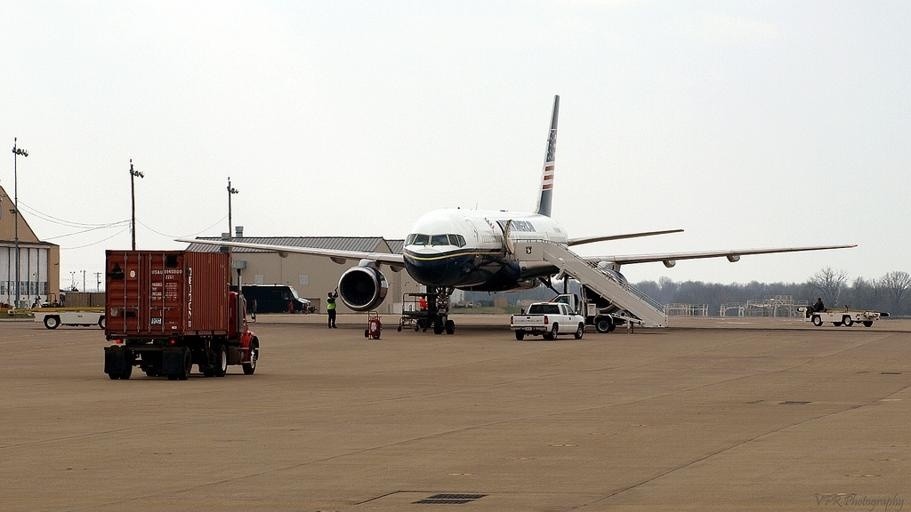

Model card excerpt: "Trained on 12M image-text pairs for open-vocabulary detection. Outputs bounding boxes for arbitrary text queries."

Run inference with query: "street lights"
[227,176,240,253]
[69,271,76,291]
[129,157,145,251]
[10,136,30,310]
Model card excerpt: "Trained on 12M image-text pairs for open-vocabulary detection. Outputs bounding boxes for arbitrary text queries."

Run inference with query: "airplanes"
[170,92,862,334]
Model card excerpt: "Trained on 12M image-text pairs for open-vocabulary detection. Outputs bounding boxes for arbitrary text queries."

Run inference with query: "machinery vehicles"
[796,305,894,328]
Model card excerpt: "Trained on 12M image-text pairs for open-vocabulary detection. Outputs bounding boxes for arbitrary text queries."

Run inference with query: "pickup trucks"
[512,300,587,341]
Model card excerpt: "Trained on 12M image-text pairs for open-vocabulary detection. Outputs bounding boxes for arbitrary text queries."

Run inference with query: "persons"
[814,297,825,311]
[326,290,338,329]
[418,296,428,311]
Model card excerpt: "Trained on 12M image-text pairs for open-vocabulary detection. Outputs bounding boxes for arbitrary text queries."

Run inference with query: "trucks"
[103,247,262,381]
[549,293,627,334]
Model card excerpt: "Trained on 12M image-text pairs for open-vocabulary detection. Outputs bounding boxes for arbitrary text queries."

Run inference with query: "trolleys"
[364,310,383,340]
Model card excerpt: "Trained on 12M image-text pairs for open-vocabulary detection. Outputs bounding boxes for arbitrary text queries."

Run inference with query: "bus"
[231,283,310,314]
